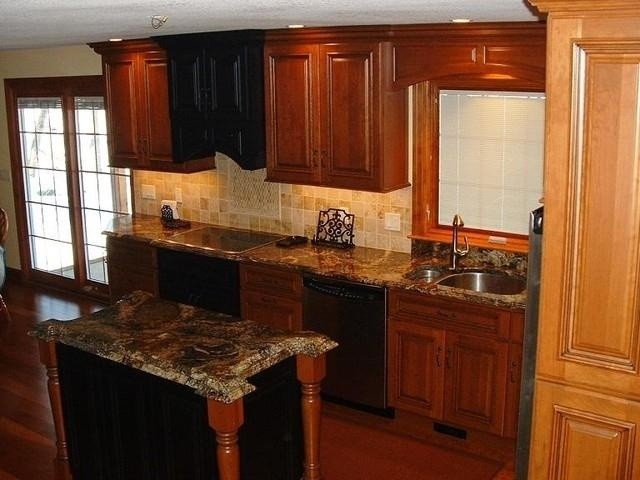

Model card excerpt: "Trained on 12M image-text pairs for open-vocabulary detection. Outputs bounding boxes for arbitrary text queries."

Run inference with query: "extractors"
[169,121,267,171]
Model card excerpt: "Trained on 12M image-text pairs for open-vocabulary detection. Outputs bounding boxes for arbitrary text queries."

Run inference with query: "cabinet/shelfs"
[387,290,525,460]
[87,34,216,173]
[263,25,411,195]
[106,237,157,306]
[240,263,302,330]
[525,0,640,480]
[149,30,266,170]
[155,247,241,320]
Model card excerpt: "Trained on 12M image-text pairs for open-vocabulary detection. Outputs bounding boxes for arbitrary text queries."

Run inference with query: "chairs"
[0,208,12,323]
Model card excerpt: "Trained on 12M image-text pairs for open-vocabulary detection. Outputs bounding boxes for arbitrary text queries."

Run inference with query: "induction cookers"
[159,224,286,255]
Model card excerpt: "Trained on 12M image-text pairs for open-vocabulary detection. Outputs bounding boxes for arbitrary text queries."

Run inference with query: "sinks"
[437,272,525,296]
[408,270,440,283]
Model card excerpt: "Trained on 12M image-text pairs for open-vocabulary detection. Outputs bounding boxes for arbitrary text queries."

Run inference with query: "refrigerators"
[511,204,546,478]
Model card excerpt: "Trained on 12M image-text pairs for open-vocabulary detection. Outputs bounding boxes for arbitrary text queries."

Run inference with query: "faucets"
[449,214,469,268]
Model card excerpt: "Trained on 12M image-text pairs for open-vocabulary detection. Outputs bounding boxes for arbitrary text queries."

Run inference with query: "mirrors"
[425,77,545,255]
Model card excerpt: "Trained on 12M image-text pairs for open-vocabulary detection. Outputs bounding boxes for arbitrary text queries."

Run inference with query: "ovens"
[298,270,397,418]
